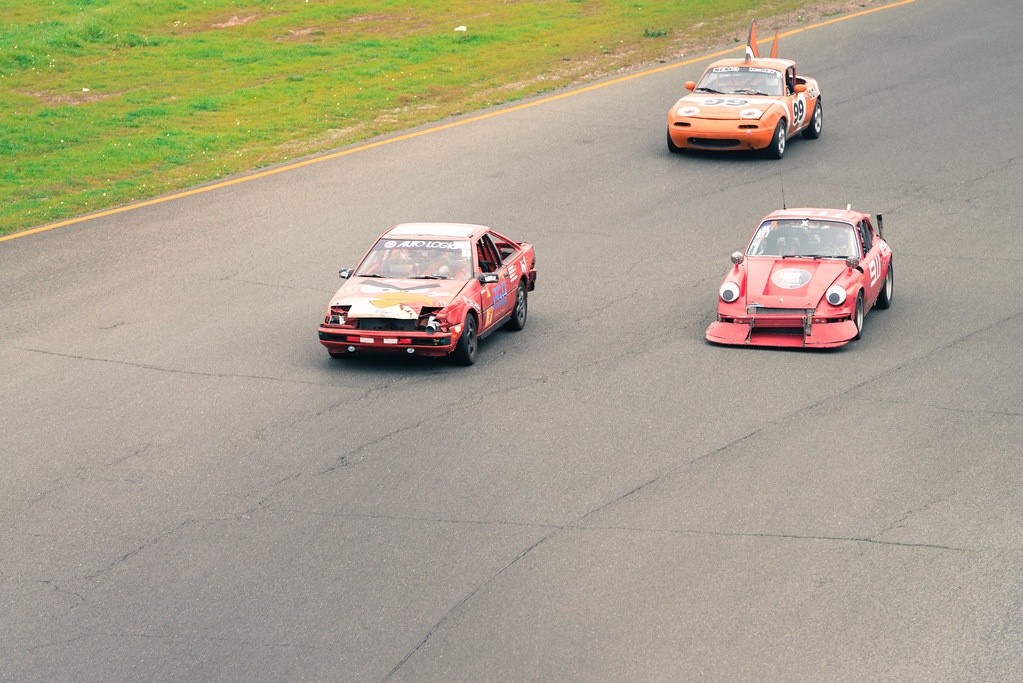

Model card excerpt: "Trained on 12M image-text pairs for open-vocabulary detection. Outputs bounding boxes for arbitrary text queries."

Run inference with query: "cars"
[318,222,537,367]
[704,207,894,351]
[666,17,823,160]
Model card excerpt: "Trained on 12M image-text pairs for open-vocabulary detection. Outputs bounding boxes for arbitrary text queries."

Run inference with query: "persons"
[826,228,850,256]
[757,74,779,94]
[425,249,468,279]
